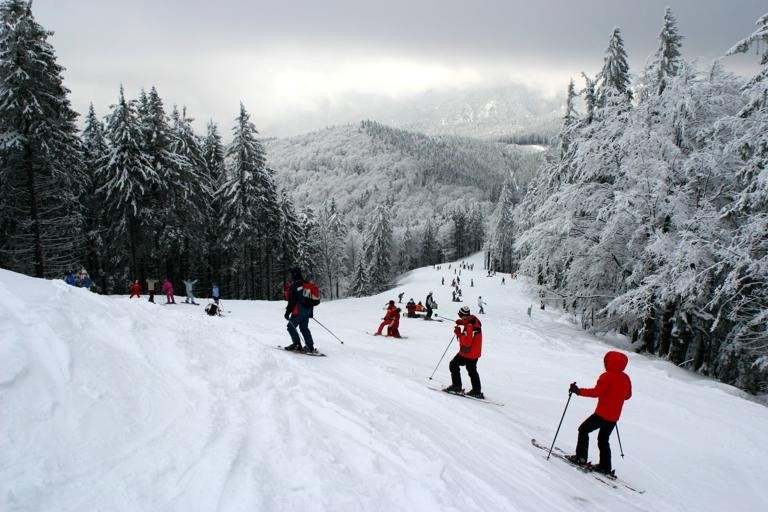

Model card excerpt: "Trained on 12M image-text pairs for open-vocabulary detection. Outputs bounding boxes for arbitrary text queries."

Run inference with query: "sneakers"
[467,390,481,395]
[568,455,586,465]
[595,463,611,473]
[302,346,313,352]
[285,344,301,350]
[447,386,462,391]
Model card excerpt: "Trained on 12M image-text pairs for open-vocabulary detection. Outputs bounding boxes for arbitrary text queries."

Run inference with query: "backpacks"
[303,283,320,306]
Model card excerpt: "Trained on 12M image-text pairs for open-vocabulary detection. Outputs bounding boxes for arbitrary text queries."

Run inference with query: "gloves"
[570,384,580,394]
[285,311,289,320]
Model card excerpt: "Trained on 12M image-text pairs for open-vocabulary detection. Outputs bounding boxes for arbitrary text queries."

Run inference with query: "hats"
[290,267,302,281]
[457,306,470,316]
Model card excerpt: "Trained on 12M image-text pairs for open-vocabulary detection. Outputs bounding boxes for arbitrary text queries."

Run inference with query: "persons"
[146,275,159,304]
[128,279,142,299]
[373,260,518,338]
[160,275,176,304]
[447,306,482,399]
[564,350,632,474]
[211,285,220,305]
[181,278,198,303]
[284,268,314,352]
